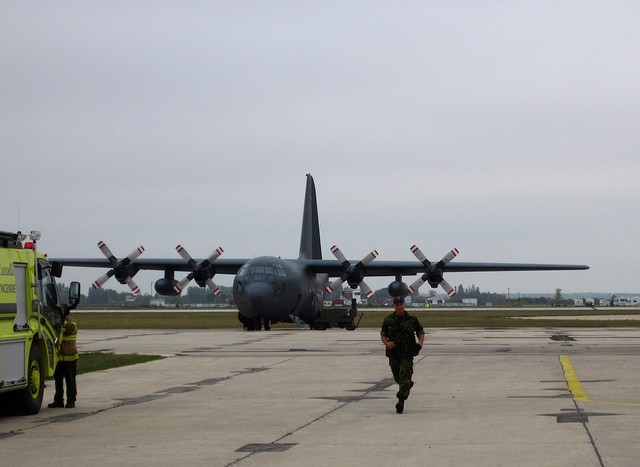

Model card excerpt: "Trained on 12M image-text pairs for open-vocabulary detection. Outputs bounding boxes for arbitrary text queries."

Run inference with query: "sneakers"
[48,399,64,407]
[65,399,75,409]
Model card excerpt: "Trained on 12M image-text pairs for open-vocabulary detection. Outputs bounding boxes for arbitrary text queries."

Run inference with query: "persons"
[48,303,79,407]
[381,296,425,412]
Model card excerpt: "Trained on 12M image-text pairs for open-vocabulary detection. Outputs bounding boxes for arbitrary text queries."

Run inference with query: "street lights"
[149,280,155,299]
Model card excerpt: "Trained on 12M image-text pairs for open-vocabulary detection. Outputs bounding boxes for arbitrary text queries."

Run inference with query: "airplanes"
[48,173,588,331]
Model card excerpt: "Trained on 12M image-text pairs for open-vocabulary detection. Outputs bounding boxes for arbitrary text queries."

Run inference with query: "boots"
[396,397,404,413]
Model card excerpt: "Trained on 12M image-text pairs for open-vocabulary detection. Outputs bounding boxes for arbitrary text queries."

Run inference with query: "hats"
[393,297,404,305]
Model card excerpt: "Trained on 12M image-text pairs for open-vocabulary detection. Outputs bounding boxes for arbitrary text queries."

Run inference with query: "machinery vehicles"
[0,231,79,416]
